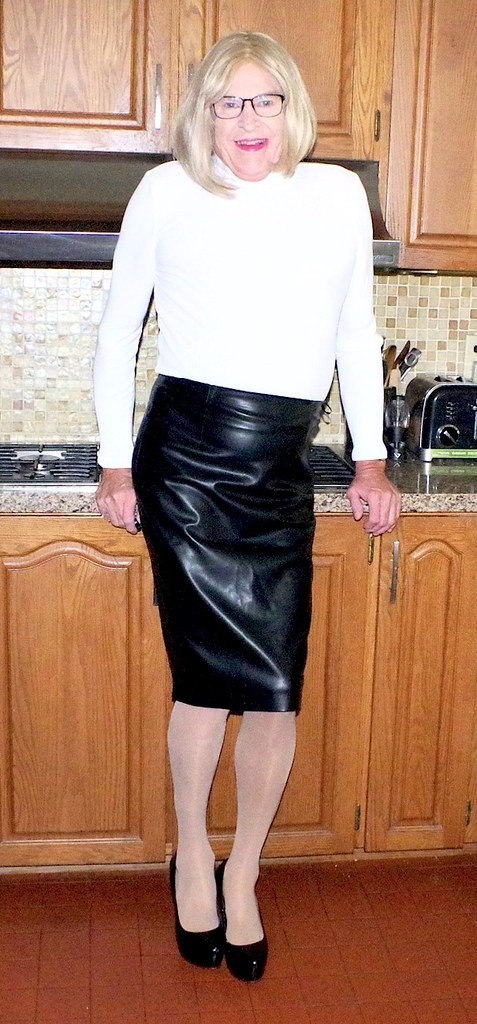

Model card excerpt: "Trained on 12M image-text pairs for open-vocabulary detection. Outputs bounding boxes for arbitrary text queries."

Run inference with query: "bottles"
[385,396,409,461]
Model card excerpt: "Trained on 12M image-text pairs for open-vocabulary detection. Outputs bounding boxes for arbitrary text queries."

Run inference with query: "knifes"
[394,340,410,365]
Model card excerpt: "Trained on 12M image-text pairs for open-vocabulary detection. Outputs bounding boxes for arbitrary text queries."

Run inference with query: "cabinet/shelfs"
[378,1,477,272]
[0,1,380,170]
[0,512,476,874]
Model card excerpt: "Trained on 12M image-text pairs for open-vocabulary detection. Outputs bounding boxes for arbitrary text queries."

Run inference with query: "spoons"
[384,345,396,387]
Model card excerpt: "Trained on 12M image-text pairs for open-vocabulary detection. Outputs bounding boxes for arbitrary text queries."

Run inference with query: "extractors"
[0,149,400,268]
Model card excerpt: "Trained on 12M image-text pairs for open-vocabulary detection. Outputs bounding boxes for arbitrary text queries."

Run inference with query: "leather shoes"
[216,857,267,981]
[170,850,224,968]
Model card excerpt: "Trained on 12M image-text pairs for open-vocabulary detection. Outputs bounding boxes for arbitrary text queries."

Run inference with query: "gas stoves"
[0,441,360,488]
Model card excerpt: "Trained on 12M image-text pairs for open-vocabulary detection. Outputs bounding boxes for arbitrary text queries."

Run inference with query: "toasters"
[404,374,477,461]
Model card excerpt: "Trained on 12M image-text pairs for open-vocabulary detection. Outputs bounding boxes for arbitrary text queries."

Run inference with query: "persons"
[93,31,405,982]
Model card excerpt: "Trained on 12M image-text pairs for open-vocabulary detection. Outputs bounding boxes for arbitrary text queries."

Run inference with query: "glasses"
[213,93,285,119]
